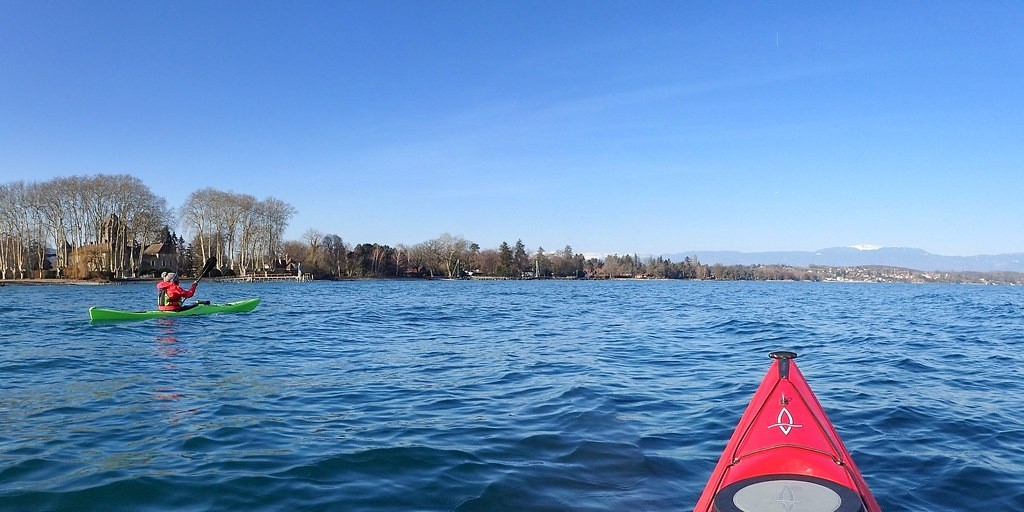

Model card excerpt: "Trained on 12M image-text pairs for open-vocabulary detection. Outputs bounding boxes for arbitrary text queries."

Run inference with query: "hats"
[161,272,178,284]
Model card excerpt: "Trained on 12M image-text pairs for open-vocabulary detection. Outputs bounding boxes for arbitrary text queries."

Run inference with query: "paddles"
[181,257,218,302]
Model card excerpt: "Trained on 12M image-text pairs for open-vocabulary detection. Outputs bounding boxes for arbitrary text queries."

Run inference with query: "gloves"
[192,281,197,285]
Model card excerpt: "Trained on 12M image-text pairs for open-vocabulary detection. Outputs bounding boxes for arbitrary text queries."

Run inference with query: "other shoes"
[202,301,210,304]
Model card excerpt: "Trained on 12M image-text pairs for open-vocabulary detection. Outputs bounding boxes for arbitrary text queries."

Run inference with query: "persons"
[156,271,210,312]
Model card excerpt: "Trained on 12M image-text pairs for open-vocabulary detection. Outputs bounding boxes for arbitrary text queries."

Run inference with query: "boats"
[88,296,262,321]
